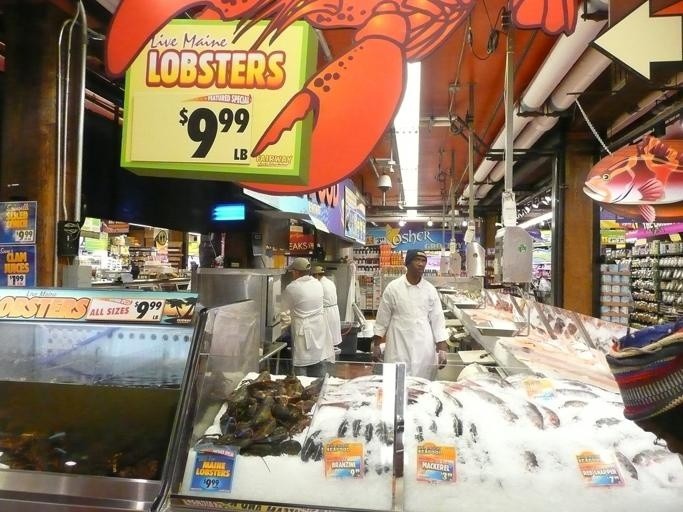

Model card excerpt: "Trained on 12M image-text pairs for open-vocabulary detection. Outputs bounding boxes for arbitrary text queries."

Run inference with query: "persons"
[278,256,326,376]
[370,248,449,381]
[310,265,342,348]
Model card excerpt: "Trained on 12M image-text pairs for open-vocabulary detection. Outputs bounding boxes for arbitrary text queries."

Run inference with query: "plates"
[457,349,496,366]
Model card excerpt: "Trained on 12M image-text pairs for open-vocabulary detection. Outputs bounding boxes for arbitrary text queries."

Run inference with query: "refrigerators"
[190,268,283,346]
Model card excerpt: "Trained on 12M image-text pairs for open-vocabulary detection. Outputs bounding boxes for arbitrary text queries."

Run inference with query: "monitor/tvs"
[208,203,254,231]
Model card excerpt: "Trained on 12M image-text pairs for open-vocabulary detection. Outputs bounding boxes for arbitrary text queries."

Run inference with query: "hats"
[284,256,311,271]
[405,247,427,263]
[311,265,325,274]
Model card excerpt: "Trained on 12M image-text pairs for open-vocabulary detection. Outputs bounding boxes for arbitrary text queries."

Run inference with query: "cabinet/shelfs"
[0,309,218,512]
[601,239,683,330]
[129,246,183,279]
[353,243,380,271]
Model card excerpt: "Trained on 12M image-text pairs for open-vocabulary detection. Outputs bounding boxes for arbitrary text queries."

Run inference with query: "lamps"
[398,216,468,227]
[516,189,551,219]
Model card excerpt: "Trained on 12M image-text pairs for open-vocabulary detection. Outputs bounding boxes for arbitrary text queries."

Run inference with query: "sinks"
[476,320,534,330]
[479,328,548,336]
[514,285,532,335]
[452,302,486,310]
[439,290,457,295]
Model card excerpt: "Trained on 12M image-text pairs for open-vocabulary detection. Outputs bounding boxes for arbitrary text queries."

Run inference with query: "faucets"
[469,275,495,306]
[448,268,459,290]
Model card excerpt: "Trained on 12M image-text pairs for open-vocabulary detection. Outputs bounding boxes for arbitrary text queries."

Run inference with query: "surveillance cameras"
[377,175,392,192]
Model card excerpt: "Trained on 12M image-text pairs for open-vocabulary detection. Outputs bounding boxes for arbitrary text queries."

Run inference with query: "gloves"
[371,346,381,362]
[438,350,447,370]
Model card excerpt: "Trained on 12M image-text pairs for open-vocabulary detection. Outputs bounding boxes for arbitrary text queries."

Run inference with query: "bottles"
[353,243,405,270]
